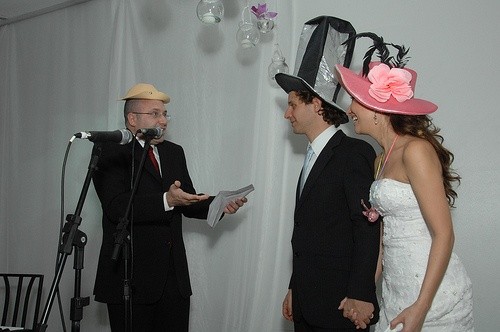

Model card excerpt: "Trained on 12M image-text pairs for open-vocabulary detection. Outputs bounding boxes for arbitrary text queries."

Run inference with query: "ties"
[297,146,315,195]
[146,144,161,176]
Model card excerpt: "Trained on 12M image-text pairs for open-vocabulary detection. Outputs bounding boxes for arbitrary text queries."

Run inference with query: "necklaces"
[374,134,397,182]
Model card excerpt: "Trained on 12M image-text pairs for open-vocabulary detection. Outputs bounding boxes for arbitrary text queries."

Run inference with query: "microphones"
[137,128,162,139]
[76,129,132,144]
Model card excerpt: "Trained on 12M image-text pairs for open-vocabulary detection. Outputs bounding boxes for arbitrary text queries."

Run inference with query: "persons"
[273,80,380,332]
[332,61,477,332]
[88,84,248,332]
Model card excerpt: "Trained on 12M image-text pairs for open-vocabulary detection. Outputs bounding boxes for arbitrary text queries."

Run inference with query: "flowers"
[250,3,278,20]
[367,63,414,102]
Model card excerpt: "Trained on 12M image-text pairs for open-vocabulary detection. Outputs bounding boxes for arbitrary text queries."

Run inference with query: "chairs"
[0,273,45,332]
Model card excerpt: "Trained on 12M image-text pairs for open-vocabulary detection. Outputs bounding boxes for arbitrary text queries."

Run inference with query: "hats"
[276,14,358,122]
[116,83,171,104]
[335,31,438,115]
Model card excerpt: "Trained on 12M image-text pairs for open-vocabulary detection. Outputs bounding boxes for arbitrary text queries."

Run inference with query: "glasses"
[126,110,171,121]
